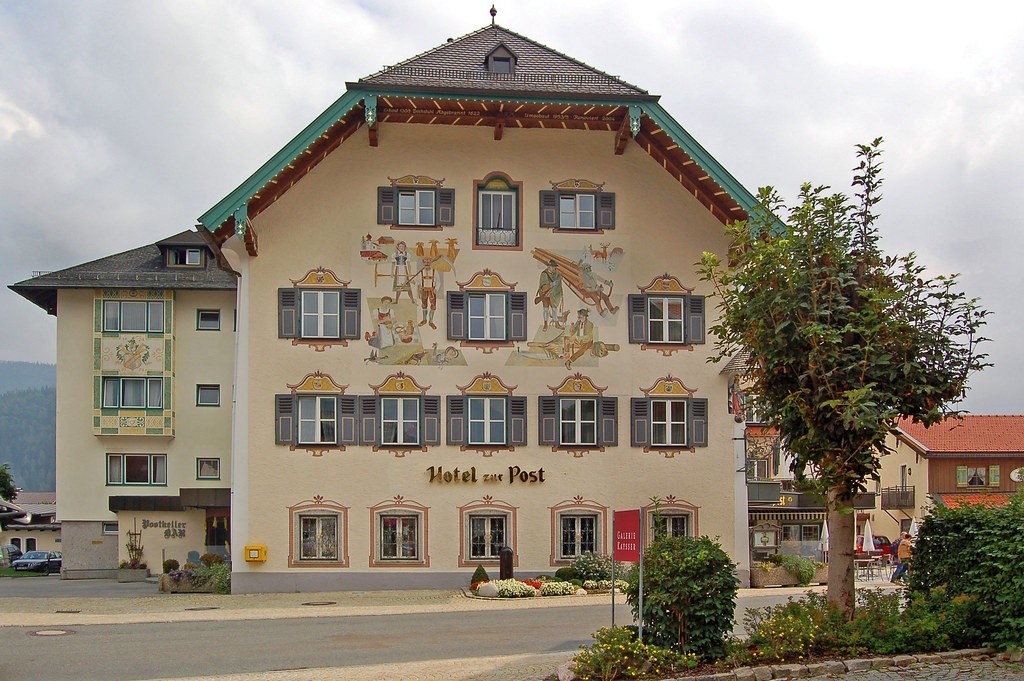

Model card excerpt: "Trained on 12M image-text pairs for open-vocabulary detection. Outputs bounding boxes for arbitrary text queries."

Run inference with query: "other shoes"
[891,579,897,583]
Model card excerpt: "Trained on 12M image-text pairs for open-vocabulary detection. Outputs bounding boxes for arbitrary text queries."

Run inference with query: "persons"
[890,531,913,583]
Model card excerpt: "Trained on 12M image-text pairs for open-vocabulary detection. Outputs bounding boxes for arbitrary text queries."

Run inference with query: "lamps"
[908,468,911,475]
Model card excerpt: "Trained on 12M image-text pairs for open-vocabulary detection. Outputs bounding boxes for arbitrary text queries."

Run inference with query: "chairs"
[857,554,897,581]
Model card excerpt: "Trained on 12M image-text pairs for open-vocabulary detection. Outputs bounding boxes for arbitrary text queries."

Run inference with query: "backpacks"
[890,539,900,554]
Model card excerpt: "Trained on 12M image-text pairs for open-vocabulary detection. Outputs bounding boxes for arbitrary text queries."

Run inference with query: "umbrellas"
[819,519,829,563]
[862,519,876,555]
[909,516,919,548]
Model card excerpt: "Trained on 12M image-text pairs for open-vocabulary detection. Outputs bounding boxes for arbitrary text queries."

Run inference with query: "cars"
[854,534,892,566]
[11,550,63,575]
[2,543,22,562]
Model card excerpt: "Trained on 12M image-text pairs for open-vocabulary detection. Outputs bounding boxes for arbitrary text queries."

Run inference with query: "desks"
[854,559,875,582]
